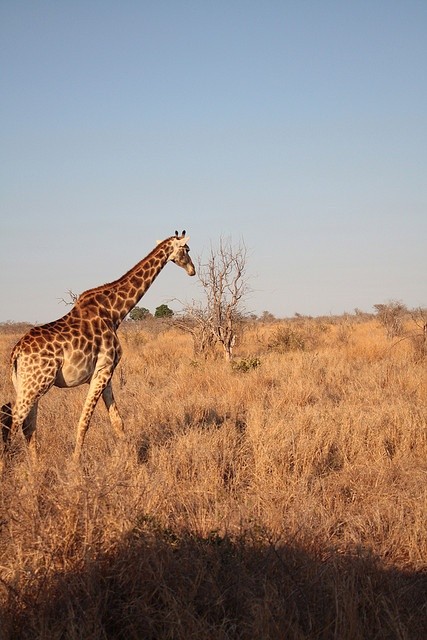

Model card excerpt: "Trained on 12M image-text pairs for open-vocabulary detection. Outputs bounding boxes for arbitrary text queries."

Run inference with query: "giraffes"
[2,230,198,470]
